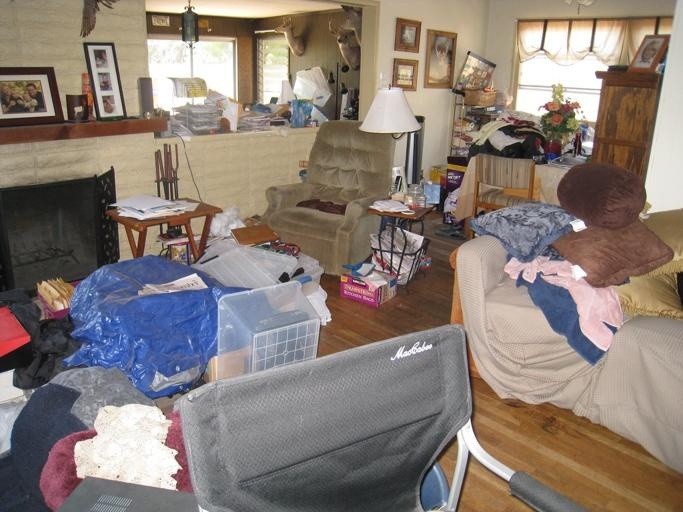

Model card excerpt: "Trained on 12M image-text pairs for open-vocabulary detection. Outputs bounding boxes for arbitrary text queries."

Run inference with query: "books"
[233,224,285,248]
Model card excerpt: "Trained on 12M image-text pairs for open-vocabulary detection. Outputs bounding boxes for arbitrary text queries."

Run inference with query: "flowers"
[537,83,587,143]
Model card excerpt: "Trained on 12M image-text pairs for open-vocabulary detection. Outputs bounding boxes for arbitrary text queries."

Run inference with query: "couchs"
[448,234,683,475]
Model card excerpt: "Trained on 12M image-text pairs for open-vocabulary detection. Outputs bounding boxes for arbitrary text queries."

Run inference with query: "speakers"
[138,76,153,119]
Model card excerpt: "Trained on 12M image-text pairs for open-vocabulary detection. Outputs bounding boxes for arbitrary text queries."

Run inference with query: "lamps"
[357,84,422,181]
[178,1,200,50]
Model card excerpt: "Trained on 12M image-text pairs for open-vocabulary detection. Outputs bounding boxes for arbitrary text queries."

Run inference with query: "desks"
[464,138,582,236]
[535,146,593,208]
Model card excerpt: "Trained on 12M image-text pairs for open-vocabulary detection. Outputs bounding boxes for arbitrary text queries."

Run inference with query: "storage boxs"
[340,273,398,308]
[292,265,326,296]
[35,281,81,320]
[216,279,321,379]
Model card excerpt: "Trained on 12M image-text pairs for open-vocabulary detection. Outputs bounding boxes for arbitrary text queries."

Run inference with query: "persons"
[1,83,46,114]
[95,51,115,112]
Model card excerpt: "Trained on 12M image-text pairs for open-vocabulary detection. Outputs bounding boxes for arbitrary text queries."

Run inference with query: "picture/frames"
[423,29,458,88]
[394,17,422,53]
[82,41,128,121]
[625,34,672,75]
[0,66,65,127]
[392,58,419,92]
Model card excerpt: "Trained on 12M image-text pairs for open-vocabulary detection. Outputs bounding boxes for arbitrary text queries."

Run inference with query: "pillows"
[470,162,683,319]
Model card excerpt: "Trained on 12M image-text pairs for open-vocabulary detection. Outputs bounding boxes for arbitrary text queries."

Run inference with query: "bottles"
[573,132,582,157]
[405,184,426,211]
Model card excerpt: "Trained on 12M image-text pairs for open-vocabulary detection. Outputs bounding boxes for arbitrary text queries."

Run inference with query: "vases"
[547,130,570,150]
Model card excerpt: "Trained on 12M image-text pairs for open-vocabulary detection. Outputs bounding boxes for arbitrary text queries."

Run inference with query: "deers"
[329,18,360,71]
[275,16,305,56]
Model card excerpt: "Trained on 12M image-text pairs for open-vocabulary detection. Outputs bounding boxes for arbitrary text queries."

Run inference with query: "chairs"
[0,364,167,512]
[173,321,587,512]
[469,153,536,240]
[259,119,408,278]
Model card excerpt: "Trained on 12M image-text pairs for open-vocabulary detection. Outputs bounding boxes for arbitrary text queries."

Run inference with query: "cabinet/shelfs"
[592,70,663,181]
